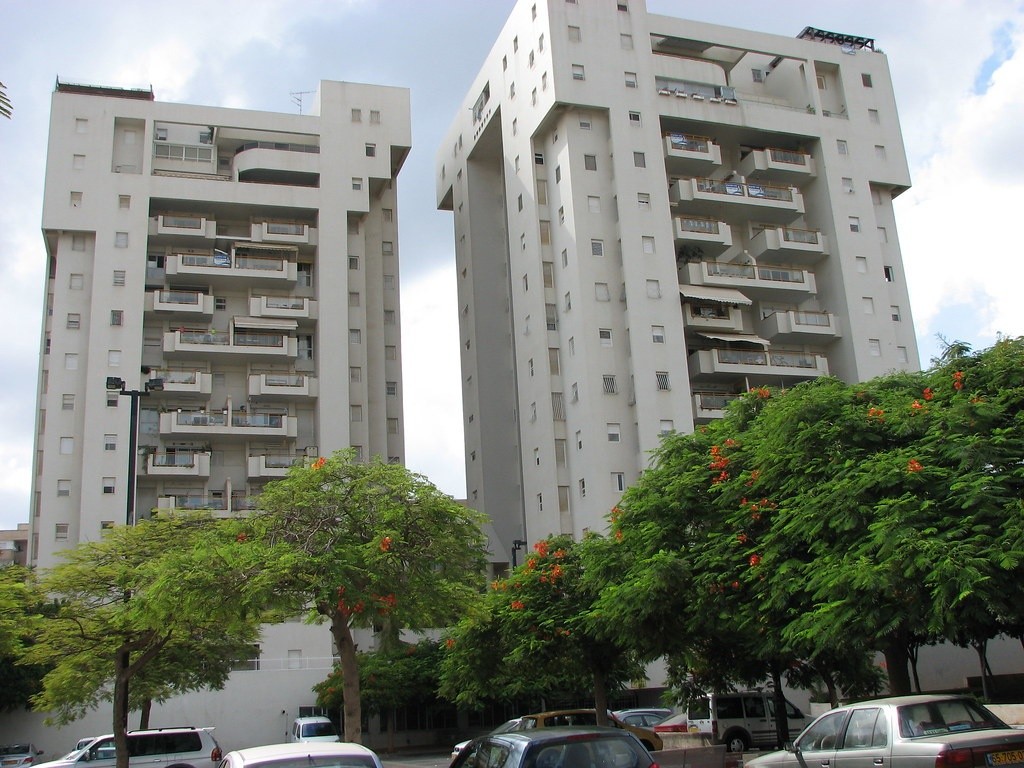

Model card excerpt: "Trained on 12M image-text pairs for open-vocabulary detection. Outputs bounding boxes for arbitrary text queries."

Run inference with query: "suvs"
[27,726,223,768]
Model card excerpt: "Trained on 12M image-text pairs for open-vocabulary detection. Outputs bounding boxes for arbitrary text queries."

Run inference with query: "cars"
[71,737,116,757]
[0,744,45,768]
[744,693,1024,768]
[218,743,384,768]
[518,709,664,753]
[451,718,522,763]
[611,708,687,734]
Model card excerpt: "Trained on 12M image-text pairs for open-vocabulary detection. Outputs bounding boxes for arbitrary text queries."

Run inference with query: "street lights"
[106,377,164,731]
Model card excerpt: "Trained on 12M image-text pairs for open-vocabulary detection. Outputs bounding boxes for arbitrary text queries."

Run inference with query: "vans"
[687,690,819,754]
[448,724,659,768]
[292,716,340,743]
